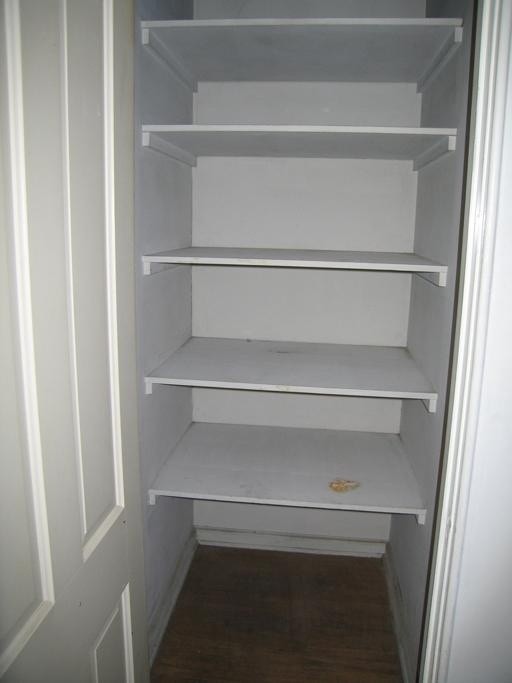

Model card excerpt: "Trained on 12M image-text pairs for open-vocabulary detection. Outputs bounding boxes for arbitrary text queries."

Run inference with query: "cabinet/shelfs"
[138,17,465,529]
